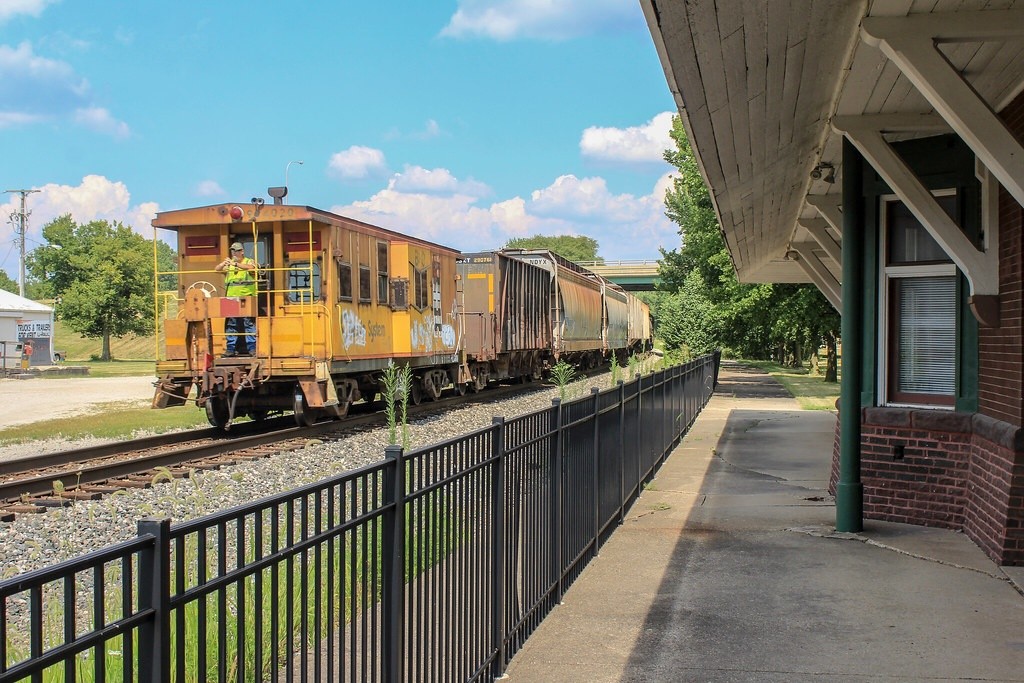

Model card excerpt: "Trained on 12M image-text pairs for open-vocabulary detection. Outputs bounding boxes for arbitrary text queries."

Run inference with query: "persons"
[215,243,261,358]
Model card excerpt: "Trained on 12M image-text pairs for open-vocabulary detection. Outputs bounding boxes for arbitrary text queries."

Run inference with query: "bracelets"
[235,262,238,266]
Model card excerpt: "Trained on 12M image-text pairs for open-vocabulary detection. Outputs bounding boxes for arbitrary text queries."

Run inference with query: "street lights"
[285,161,303,204]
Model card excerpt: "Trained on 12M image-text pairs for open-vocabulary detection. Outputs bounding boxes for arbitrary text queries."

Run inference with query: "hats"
[229,242,243,251]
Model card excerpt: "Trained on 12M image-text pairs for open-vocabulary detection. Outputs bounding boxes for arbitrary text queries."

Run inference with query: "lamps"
[823,165,835,184]
[810,166,821,180]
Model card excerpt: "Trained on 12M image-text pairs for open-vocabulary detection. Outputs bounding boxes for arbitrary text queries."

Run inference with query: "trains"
[151,186,654,430]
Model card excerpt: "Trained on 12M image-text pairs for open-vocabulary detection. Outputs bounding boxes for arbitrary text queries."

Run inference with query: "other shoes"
[249,349,256,357]
[221,351,235,358]
